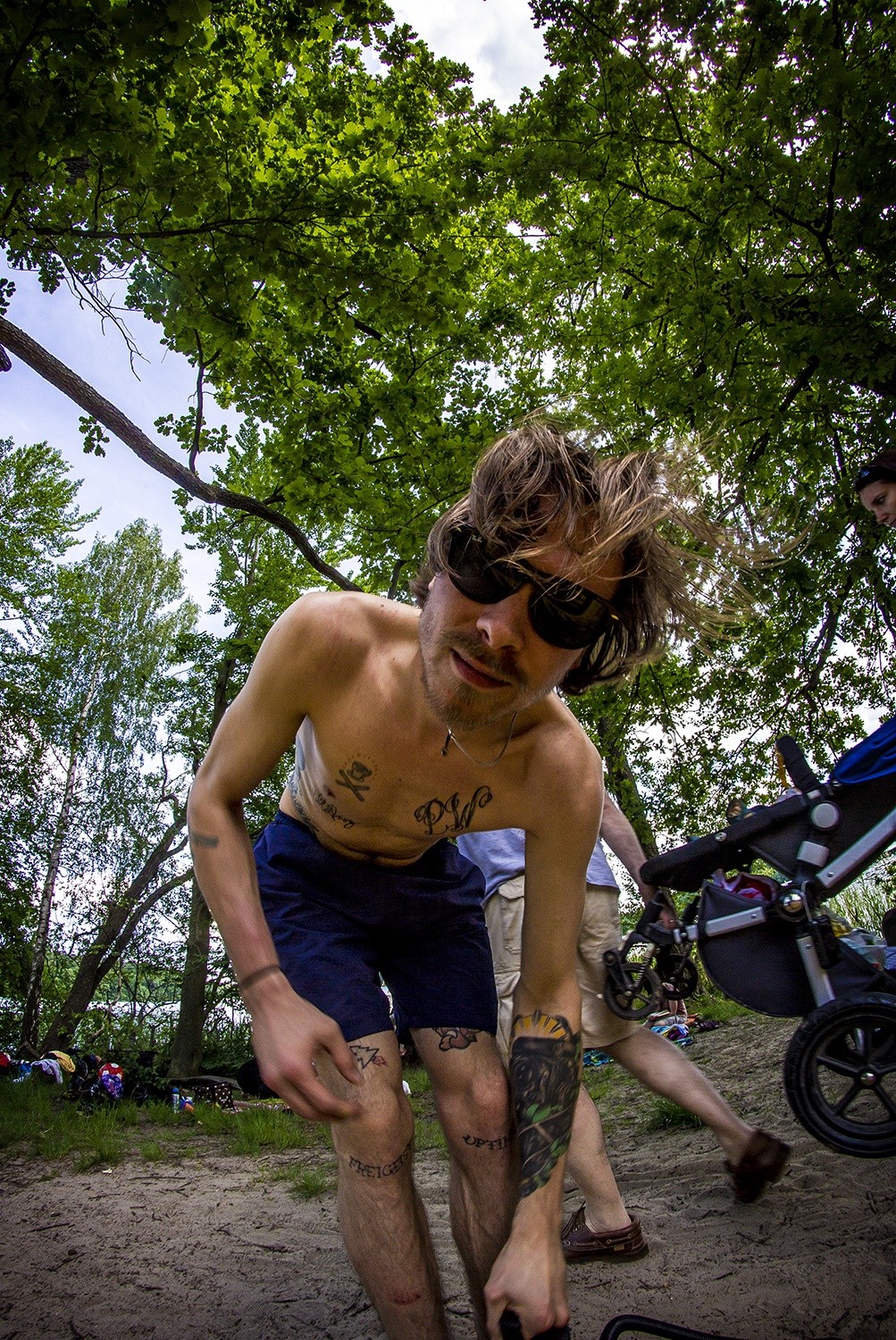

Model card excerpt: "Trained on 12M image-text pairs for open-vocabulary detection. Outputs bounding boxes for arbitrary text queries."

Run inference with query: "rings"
[670,920,674,922]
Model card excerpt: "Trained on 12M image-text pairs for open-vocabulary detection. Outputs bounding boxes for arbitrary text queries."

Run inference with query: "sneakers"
[559,1200,650,1266]
[723,1128,792,1205]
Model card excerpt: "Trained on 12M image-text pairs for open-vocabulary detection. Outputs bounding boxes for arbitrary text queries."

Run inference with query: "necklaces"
[442,710,518,768]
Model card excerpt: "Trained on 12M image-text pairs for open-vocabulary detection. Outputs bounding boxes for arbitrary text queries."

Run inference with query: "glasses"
[445,523,622,651]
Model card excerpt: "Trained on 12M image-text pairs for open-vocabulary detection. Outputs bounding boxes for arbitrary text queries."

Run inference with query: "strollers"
[601,709,896,1161]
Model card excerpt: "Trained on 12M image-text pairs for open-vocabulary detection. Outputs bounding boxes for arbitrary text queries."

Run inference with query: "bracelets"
[240,964,281,988]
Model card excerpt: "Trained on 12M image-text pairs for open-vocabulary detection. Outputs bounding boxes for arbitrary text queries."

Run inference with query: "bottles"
[820,904,853,936]
[171,1088,180,1113]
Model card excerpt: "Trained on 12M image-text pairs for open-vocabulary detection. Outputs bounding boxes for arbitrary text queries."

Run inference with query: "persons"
[457,785,794,1264]
[643,983,688,1028]
[855,448,896,528]
[186,422,737,1339]
